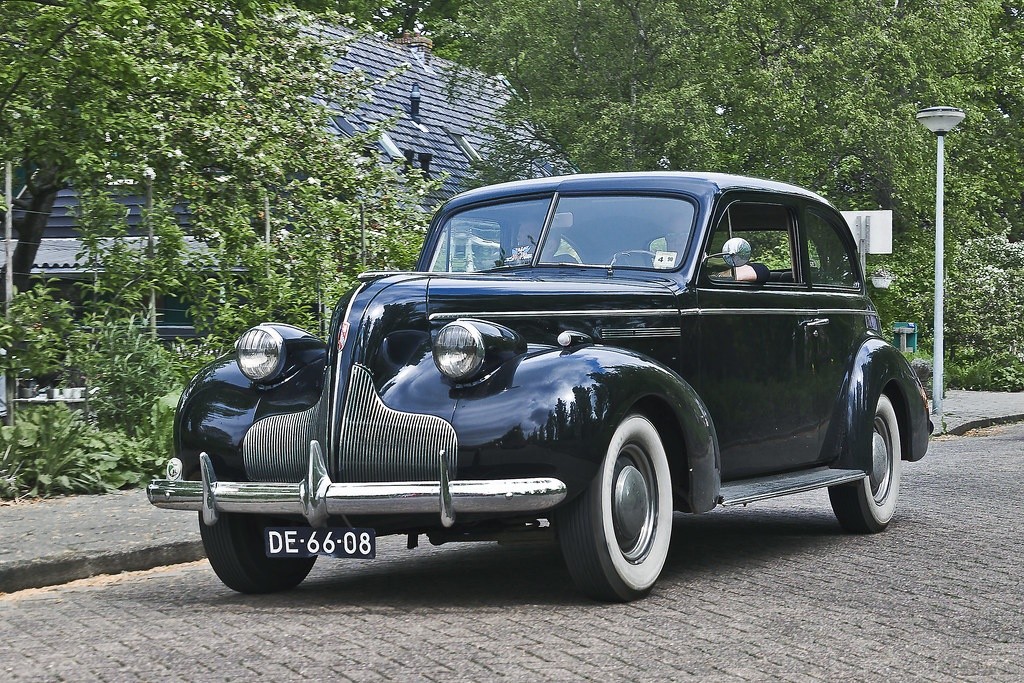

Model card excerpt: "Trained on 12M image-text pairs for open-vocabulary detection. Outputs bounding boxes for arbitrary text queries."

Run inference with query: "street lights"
[917,106,966,436]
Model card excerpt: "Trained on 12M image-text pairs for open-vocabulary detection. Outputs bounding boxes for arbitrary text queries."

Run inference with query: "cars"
[146,168,935,603]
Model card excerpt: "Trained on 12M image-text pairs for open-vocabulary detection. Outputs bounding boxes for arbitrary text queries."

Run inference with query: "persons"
[517,214,580,264]
[664,231,770,282]
